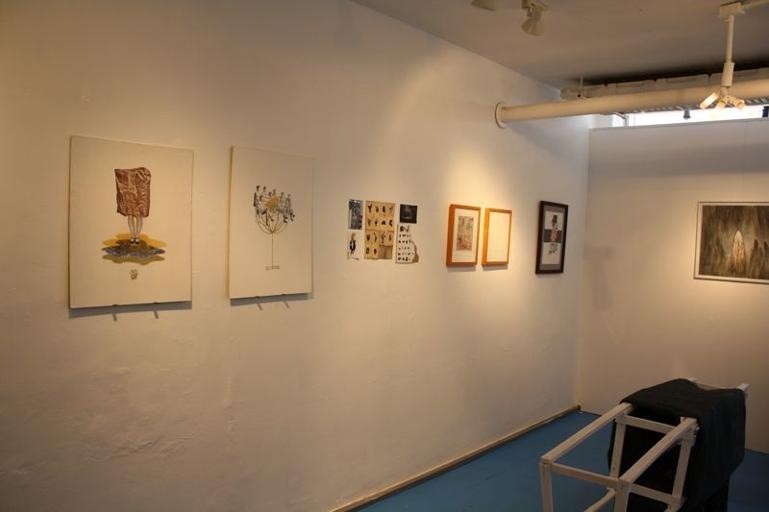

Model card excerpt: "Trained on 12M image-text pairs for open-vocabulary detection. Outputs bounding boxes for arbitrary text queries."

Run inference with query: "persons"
[253,184,296,226]
[126,215,142,245]
[699,205,769,280]
[401,205,414,222]
[348,199,363,258]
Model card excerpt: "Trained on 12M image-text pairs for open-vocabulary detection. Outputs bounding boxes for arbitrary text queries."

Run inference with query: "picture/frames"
[692,202,768,286]
[536,200,569,276]
[447,205,481,268]
[482,209,513,267]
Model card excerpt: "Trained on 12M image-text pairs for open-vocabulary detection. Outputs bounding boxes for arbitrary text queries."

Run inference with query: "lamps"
[519,0,548,37]
[469,1,501,12]
